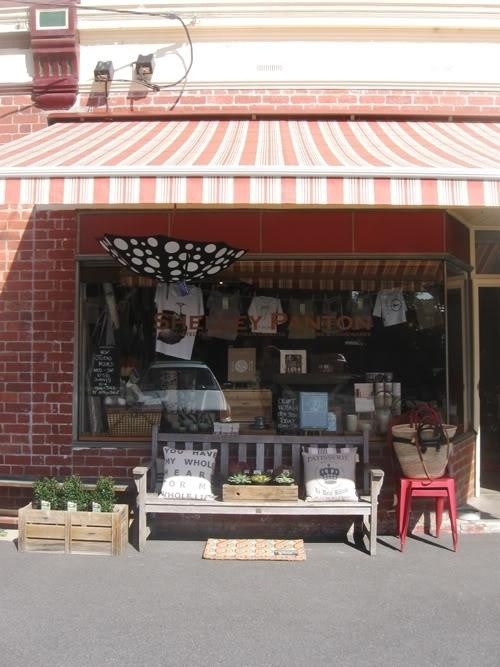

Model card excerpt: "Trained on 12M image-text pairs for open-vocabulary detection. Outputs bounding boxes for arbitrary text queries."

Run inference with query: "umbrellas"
[95,214,248,285]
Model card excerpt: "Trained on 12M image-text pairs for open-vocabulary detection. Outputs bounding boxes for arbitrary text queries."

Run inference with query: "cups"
[347,415,357,432]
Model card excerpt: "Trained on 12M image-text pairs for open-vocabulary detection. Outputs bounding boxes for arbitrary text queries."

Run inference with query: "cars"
[138,359,232,425]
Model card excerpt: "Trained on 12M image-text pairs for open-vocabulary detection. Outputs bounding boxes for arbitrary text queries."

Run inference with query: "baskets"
[103,405,163,437]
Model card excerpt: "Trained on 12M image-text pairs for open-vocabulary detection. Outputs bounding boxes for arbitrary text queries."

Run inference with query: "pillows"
[302,452,361,503]
[161,446,218,502]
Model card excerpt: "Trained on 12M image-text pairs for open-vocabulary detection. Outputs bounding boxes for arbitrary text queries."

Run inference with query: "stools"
[397,475,459,553]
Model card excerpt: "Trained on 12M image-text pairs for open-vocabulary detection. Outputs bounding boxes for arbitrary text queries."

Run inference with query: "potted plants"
[222,473,298,501]
[17,473,129,556]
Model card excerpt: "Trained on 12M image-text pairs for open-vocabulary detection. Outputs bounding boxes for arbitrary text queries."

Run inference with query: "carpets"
[203,537,307,559]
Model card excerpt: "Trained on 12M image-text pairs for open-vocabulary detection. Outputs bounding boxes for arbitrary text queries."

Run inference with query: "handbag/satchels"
[392,423,457,479]
[86,344,120,396]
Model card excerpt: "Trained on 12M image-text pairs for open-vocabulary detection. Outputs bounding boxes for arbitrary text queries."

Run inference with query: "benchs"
[133,424,385,556]
